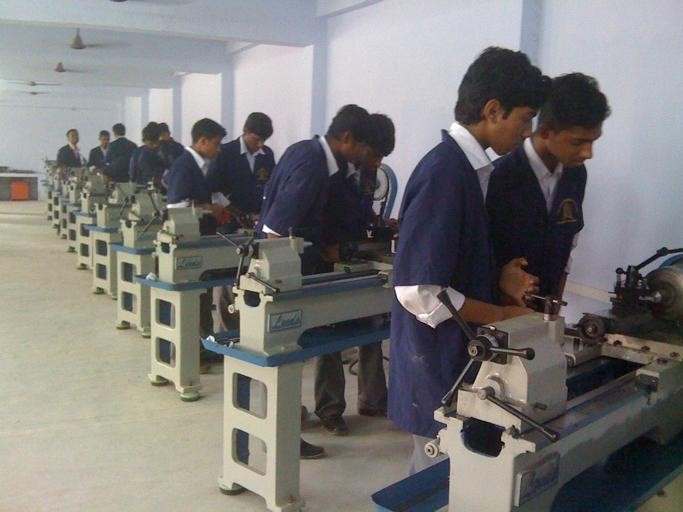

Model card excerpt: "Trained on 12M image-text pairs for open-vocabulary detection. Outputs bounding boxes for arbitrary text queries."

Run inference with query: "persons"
[56,129,81,167]
[384,47,554,474]
[252,104,373,462]
[100,123,138,182]
[487,72,613,319]
[167,117,227,373]
[89,130,110,165]
[209,111,277,362]
[128,121,185,196]
[313,112,396,437]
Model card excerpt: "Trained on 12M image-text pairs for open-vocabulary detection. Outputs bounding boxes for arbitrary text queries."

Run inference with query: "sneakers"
[357,408,388,418]
[320,416,350,435]
[300,440,324,459]
[200,363,209,375]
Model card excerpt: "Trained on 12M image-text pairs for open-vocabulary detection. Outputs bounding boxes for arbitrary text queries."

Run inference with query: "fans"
[0,0,128,96]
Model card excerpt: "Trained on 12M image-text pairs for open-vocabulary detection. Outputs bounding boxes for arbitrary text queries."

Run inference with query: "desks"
[1,172,41,200]
